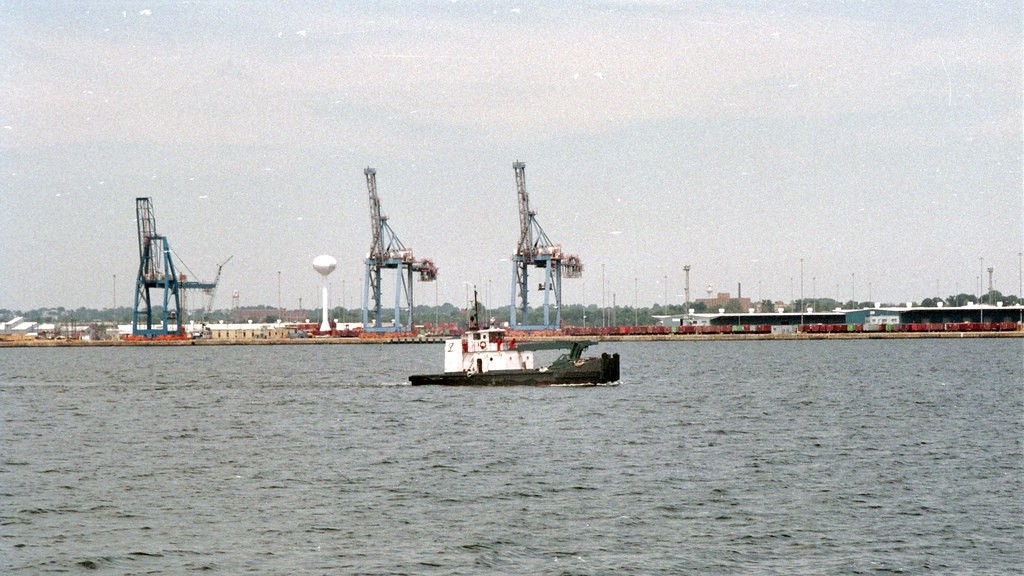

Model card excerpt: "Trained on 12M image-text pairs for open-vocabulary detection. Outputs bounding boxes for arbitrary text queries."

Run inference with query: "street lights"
[112,274,117,322]
[601,263,605,327]
[635,277,638,326]
[801,259,805,333]
[1018,252,1023,327]
[980,257,984,331]
[277,271,282,323]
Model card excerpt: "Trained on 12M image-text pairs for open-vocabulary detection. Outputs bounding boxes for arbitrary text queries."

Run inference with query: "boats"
[408,284,620,386]
